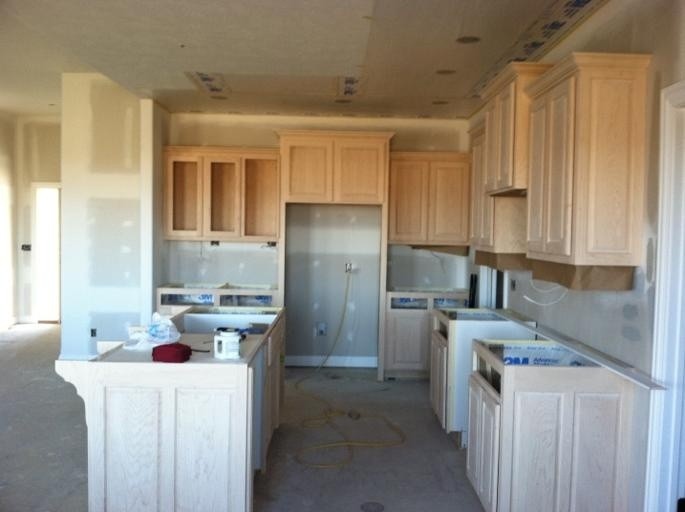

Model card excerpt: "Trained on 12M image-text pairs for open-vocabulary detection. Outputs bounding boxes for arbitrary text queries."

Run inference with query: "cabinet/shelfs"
[527,51,654,267]
[430,308,537,432]
[479,62,549,196]
[469,112,527,254]
[277,128,389,205]
[163,147,278,243]
[387,152,471,245]
[385,292,468,381]
[465,340,634,512]
[158,287,278,310]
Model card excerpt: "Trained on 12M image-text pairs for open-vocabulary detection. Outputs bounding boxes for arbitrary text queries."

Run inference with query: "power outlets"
[345,263,358,271]
[315,322,326,336]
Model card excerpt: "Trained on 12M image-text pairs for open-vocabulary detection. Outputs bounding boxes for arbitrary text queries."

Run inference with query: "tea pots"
[214,331,242,359]
